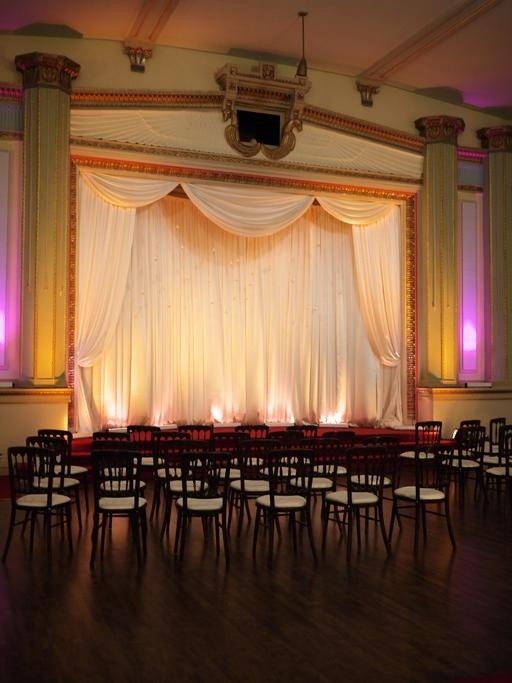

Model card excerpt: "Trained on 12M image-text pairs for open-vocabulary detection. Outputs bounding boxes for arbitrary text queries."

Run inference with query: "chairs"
[387,442,457,562]
[25,435,83,539]
[2,446,74,578]
[173,450,232,573]
[226,437,283,538]
[24,416,512,512]
[322,445,398,569]
[90,450,149,570]
[159,439,218,543]
[334,434,405,546]
[251,448,319,574]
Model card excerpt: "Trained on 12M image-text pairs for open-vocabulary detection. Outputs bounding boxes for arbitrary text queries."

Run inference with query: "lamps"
[296,10,309,78]
